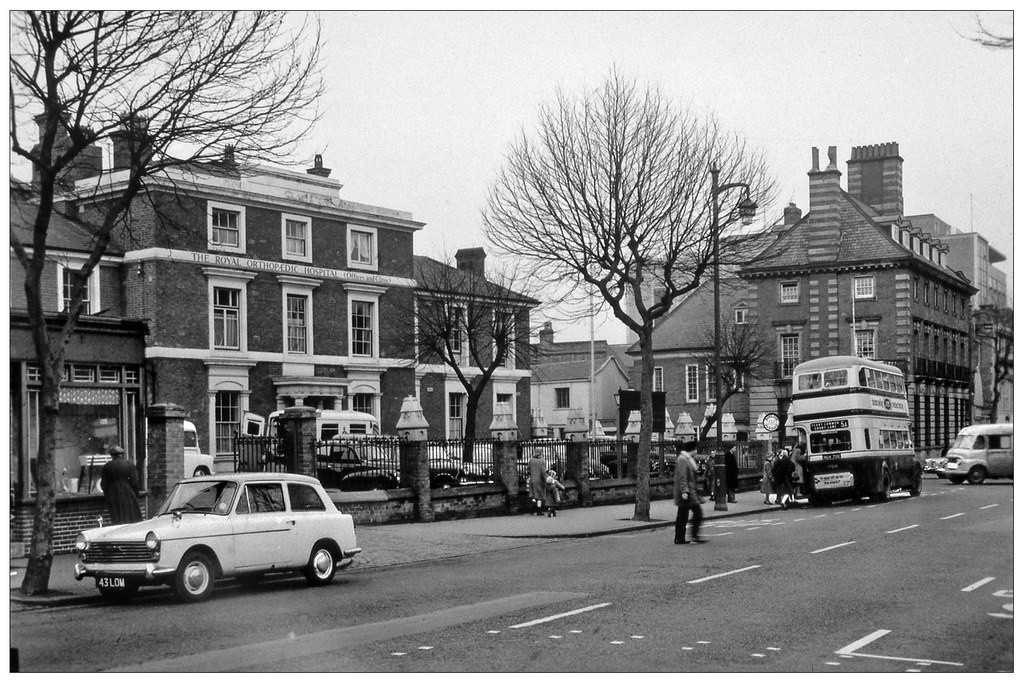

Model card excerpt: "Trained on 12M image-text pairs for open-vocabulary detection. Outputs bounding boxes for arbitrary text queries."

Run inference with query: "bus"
[791,356,923,508]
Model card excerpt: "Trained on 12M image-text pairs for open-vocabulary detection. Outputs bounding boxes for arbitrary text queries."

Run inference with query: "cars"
[73,471,363,605]
[266,426,710,494]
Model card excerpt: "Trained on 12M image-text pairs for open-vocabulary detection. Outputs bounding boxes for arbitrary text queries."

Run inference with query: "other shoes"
[553,514,556,516]
[548,514,551,517]
[690,535,709,543]
[537,512,544,516]
[728,500,738,503]
[780,502,788,509]
[674,539,690,544]
[531,511,534,515]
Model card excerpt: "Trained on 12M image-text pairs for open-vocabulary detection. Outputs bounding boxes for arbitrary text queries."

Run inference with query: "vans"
[935,422,1014,486]
[182,420,216,478]
[241,407,381,459]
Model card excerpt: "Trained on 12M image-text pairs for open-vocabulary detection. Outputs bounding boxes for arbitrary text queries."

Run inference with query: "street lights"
[707,160,763,512]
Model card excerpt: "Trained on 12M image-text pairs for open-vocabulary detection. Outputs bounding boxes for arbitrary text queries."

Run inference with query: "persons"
[526,447,565,517]
[761,441,807,506]
[673,440,707,543]
[725,444,738,502]
[703,450,715,500]
[101,444,142,523]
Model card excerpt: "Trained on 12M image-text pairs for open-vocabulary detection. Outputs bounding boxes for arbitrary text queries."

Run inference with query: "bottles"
[70,478,79,493]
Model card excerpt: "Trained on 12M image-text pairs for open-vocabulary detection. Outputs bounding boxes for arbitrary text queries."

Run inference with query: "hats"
[109,446,124,455]
